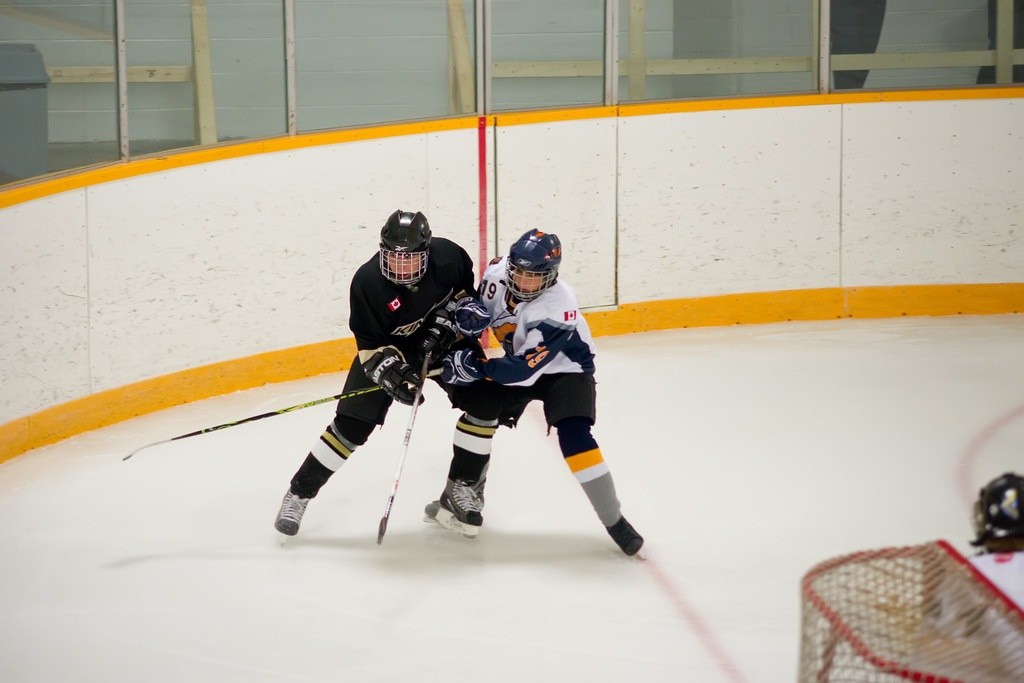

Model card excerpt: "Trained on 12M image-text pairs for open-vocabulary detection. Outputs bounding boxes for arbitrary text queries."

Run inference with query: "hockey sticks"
[120,367,445,463]
[375,350,435,546]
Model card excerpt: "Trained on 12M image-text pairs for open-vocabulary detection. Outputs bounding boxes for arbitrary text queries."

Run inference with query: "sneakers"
[423,476,484,523]
[423,478,482,539]
[606,516,644,556]
[274,488,308,544]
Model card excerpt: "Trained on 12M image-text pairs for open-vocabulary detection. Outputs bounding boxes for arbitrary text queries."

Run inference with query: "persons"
[275,207,506,544]
[831,0,1024,89]
[937,472,1024,683]
[437,229,643,556]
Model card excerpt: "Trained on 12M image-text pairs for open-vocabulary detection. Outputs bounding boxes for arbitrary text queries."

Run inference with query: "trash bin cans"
[0,42,58,188]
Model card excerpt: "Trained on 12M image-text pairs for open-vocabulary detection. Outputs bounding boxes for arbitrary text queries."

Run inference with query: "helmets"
[504,228,561,301]
[967,472,1024,553]
[379,209,432,286]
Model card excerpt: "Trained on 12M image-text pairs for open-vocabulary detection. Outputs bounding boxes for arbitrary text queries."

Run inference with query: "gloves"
[363,348,425,406]
[439,349,484,385]
[455,296,490,336]
[421,312,457,366]
